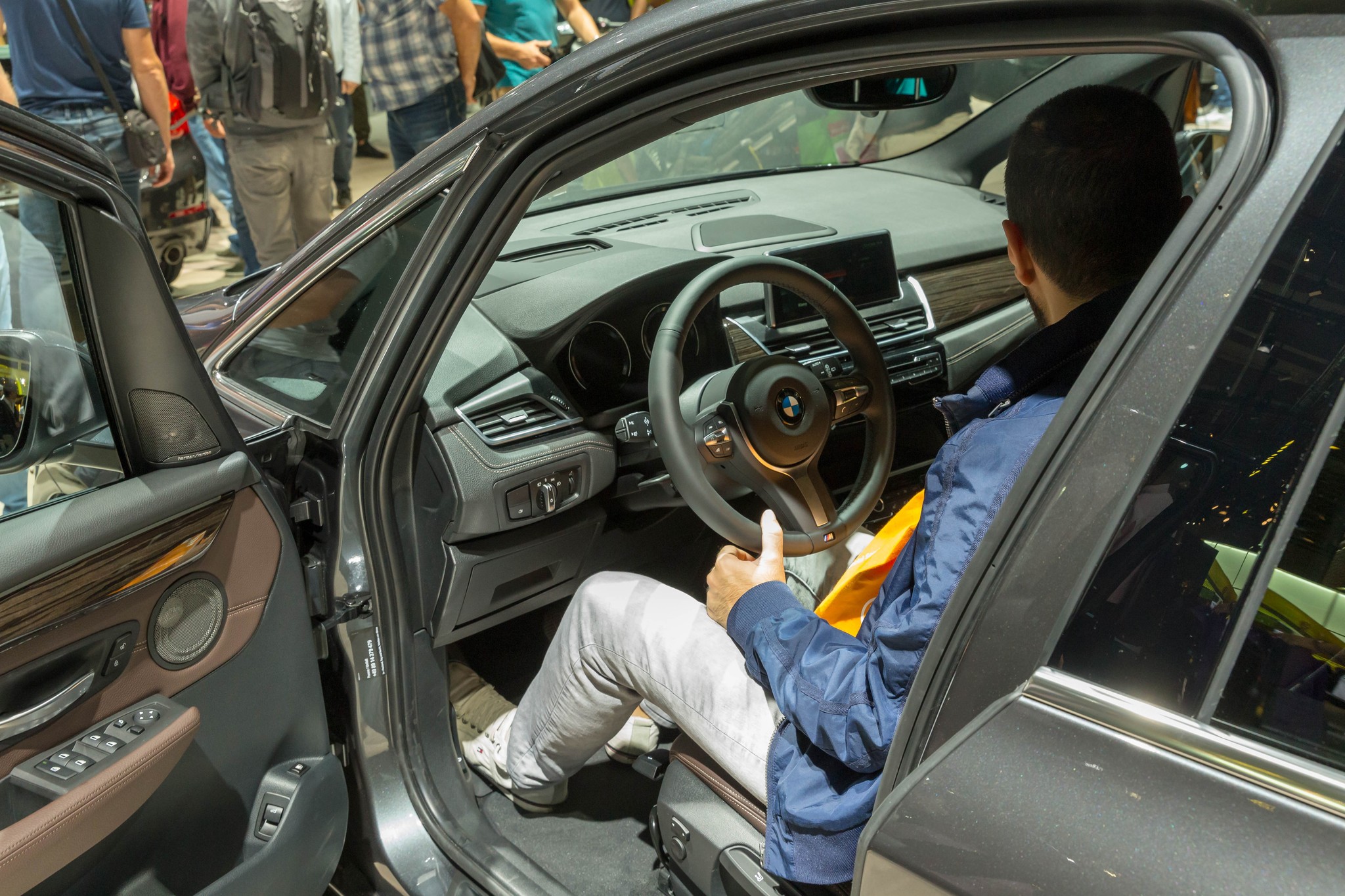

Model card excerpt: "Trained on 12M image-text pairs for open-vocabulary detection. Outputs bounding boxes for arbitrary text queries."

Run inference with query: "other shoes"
[225,260,248,277]
[338,197,352,209]
[216,249,241,262]
[355,142,389,158]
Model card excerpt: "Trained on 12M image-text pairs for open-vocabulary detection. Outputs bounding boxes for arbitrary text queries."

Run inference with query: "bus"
[0,355,30,397]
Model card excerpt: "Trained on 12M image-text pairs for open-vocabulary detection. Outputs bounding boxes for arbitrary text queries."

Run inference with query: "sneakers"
[604,717,660,764]
[442,659,568,811]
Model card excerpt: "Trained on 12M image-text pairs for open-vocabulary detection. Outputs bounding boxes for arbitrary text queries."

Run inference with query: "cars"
[0,0,1345,896]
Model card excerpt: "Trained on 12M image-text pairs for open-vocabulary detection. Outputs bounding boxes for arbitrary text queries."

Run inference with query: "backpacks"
[208,1,339,136]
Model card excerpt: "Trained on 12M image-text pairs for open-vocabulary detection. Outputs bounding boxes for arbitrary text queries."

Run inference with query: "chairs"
[651,732,785,896]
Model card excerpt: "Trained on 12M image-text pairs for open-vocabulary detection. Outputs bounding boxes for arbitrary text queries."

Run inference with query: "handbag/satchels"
[470,19,508,99]
[122,109,167,169]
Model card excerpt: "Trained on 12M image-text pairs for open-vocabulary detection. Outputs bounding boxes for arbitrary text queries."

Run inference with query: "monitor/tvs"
[763,229,902,329]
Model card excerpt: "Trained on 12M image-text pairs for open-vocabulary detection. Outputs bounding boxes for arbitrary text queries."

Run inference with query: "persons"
[0,383,27,458]
[445,82,1190,888]
[0,0,670,345]
